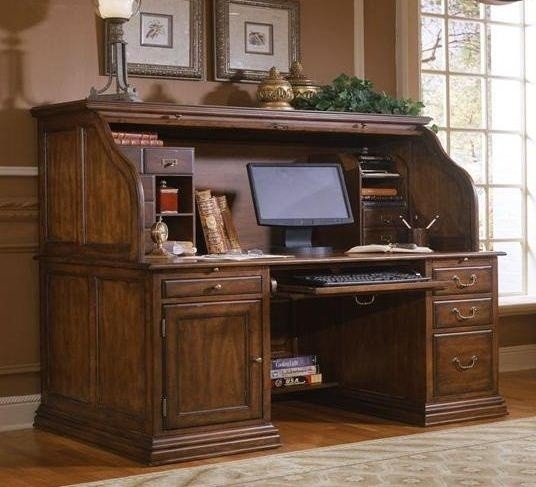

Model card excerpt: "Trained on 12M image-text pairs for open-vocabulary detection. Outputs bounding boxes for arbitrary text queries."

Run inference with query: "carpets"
[69,415,536,487]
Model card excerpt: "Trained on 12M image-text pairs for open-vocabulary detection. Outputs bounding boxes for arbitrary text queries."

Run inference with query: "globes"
[147,215,169,256]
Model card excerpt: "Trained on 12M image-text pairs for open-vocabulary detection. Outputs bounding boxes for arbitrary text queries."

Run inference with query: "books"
[194,188,225,254]
[110,128,160,139]
[210,196,232,250]
[361,184,398,195]
[362,194,403,200]
[269,366,321,379]
[269,349,314,369]
[113,138,164,147]
[217,193,241,249]
[271,373,322,389]
[160,239,197,256]
[362,200,408,207]
[346,242,435,254]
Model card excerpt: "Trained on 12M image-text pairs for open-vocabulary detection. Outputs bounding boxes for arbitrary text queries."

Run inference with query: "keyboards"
[284,269,433,287]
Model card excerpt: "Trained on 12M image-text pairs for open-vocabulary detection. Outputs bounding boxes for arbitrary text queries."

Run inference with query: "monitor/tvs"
[246,162,354,255]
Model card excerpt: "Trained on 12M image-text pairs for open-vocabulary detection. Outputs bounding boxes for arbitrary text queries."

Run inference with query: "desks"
[31,98,512,469]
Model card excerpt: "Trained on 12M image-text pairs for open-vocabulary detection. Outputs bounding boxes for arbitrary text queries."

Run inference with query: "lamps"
[87,0,143,104]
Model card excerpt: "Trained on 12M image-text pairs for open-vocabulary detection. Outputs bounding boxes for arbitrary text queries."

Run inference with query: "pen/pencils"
[426,214,440,230]
[400,215,412,230]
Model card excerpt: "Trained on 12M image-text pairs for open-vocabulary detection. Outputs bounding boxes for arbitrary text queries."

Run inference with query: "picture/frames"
[96,0,301,85]
